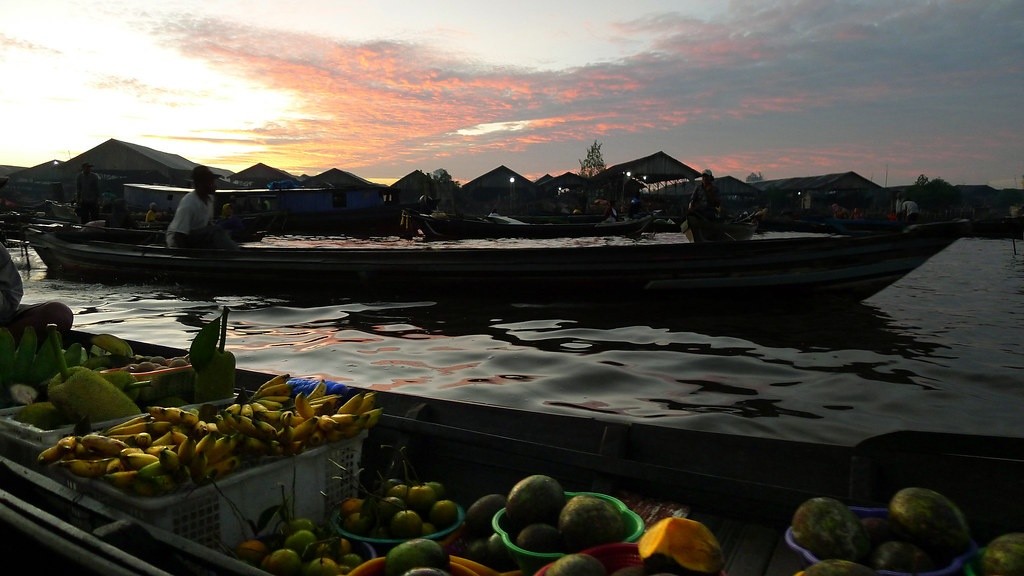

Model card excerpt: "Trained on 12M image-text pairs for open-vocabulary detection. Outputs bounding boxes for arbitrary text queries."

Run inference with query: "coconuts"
[789,487,1024,576]
[382,473,725,576]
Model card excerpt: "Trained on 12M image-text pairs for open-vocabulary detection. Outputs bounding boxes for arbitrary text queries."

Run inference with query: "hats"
[191,166,222,179]
[0,178,8,188]
[81,160,95,167]
[703,170,714,182]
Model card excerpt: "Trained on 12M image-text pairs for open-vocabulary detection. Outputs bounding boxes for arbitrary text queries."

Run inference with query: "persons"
[146,203,157,223]
[76,163,102,224]
[689,169,723,215]
[0,242,73,351]
[897,192,904,220]
[630,192,642,219]
[165,167,239,248]
[901,198,919,223]
[227,200,239,221]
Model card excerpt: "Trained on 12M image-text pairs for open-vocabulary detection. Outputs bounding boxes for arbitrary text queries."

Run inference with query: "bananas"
[36,375,383,496]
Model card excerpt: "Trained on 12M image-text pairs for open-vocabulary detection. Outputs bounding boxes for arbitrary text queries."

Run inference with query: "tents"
[0,137,1023,215]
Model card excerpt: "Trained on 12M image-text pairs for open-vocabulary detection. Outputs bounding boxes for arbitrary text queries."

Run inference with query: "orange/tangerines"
[233,478,458,576]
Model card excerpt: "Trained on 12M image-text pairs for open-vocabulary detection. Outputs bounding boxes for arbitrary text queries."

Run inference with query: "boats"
[19,218,968,305]
[401,208,665,238]
[1,310,1024,575]
